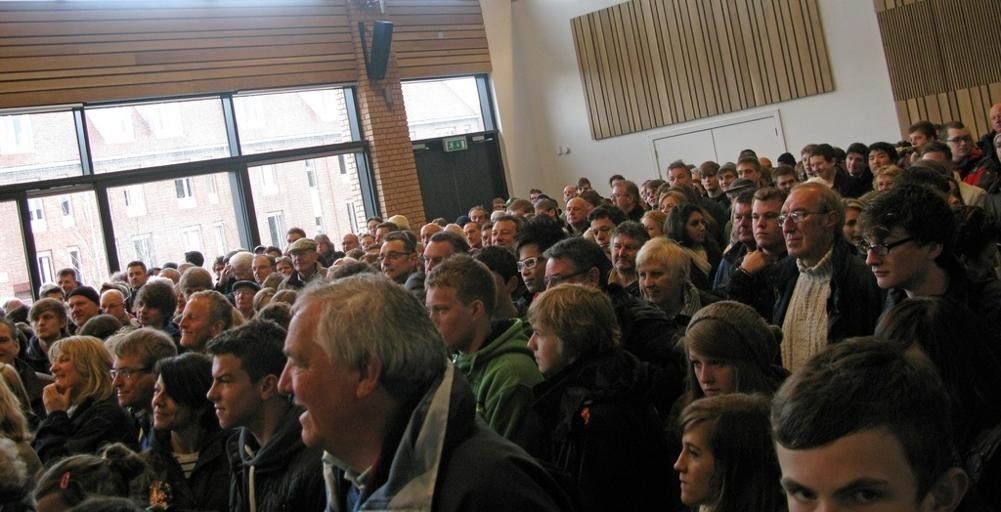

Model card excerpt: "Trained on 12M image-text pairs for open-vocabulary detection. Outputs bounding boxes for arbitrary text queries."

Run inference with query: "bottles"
[287,237,317,255]
[685,299,786,372]
[231,280,260,293]
[65,286,100,308]
[722,177,757,193]
[386,215,411,230]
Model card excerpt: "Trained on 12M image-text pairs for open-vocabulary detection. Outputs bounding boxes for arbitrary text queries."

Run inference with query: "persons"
[0,104,1000,511]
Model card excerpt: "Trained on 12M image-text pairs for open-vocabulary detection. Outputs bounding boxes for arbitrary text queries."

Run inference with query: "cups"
[542,269,582,288]
[775,211,824,228]
[860,237,914,258]
[947,134,970,144]
[377,250,410,261]
[515,256,547,273]
[106,365,152,380]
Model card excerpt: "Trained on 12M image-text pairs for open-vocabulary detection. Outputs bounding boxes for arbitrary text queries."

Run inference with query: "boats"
[370,20,393,81]
